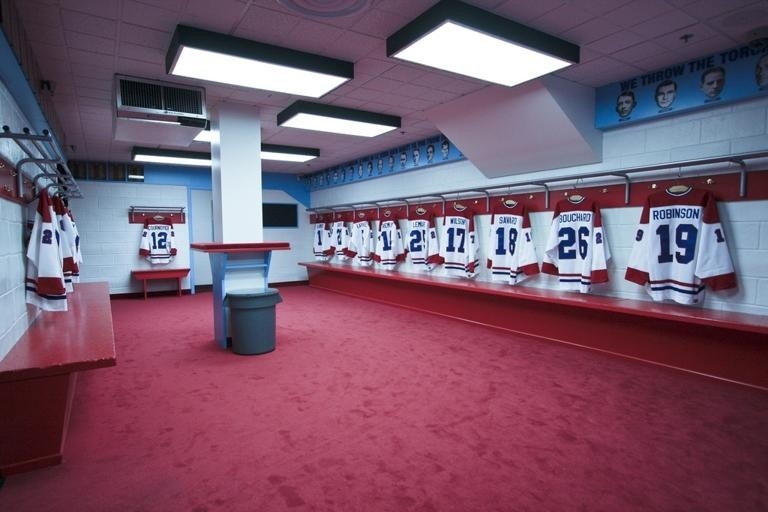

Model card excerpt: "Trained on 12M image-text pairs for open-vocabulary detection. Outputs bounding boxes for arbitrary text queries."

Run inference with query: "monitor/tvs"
[262,203,297,228]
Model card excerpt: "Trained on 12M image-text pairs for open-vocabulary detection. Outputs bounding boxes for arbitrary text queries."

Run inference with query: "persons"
[698,66,725,98]
[654,79,678,108]
[615,91,636,118]
[755,53,767,86]
[302,140,449,189]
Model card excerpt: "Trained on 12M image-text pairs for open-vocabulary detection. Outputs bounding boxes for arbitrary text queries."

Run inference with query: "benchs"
[1,279,118,477]
[132,268,193,300]
[298,256,768,397]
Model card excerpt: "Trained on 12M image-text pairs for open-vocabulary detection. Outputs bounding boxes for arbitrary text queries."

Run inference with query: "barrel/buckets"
[224,286,283,356]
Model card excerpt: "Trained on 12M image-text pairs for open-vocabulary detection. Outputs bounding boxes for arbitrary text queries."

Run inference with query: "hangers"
[310,162,690,227]
[143,209,174,228]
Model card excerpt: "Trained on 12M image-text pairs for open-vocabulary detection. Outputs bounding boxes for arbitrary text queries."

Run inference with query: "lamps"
[385,0,579,89]
[192,119,211,143]
[132,146,212,167]
[164,23,355,100]
[260,143,321,163]
[277,100,401,138]
